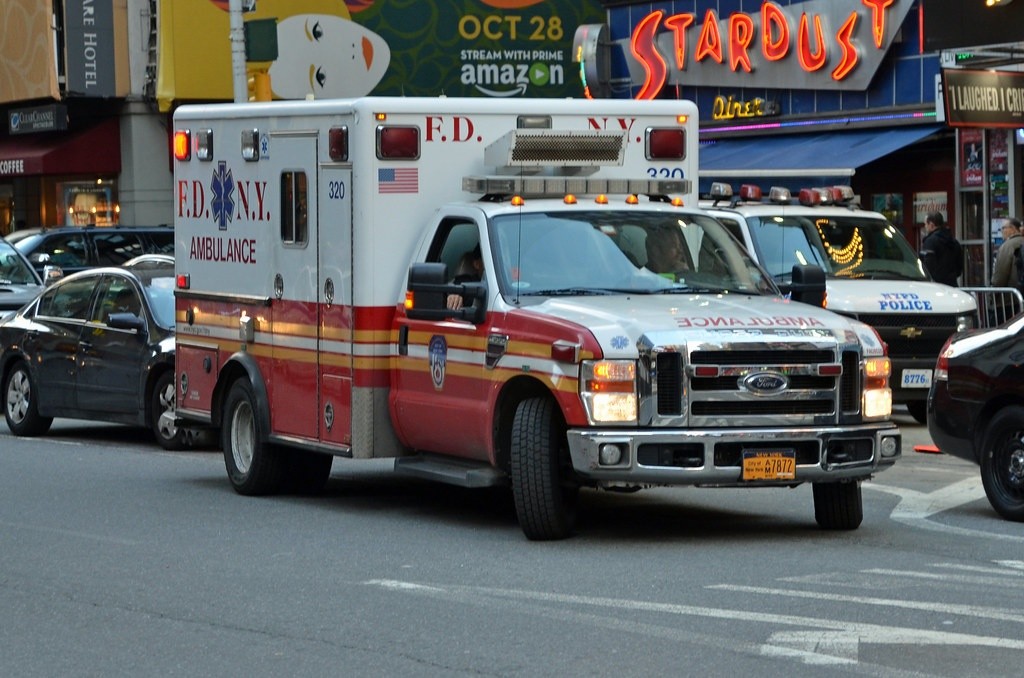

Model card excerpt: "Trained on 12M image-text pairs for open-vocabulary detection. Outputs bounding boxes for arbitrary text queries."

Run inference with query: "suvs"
[700,181,980,424]
[0,226,175,285]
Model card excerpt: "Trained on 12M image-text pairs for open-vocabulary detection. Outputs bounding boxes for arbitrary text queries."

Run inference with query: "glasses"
[1002,226,1015,230]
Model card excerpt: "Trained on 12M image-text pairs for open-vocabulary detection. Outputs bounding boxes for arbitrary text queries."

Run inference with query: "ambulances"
[175,99,905,540]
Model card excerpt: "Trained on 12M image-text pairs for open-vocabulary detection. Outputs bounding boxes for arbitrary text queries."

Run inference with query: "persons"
[881,193,897,223]
[635,229,691,291]
[446,242,484,310]
[991,217,1024,320]
[919,210,963,288]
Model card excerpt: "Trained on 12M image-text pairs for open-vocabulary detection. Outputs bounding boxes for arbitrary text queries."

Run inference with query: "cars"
[6,229,50,243]
[0,265,219,451]
[126,251,176,268]
[928,311,1024,522]
[0,237,66,317]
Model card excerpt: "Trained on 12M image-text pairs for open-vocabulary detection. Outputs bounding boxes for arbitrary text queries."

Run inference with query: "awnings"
[697,122,947,192]
[0,122,122,175]
